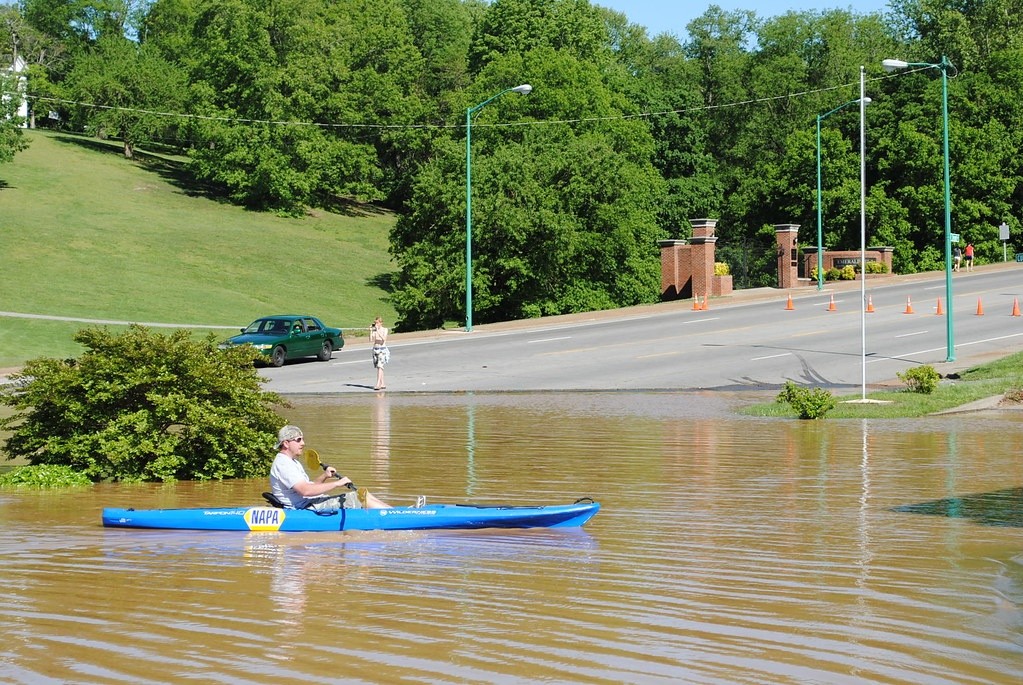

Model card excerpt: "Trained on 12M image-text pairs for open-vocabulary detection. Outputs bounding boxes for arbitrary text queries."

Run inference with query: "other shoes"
[381,385,386,389]
[374,385,381,390]
[409,495,426,509]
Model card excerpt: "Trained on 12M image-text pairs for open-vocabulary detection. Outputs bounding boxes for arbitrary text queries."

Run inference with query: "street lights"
[883,58,956,361]
[465,82,533,333]
[815,98,873,288]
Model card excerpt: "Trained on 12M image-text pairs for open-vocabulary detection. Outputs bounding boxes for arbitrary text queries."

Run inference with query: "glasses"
[287,438,304,443]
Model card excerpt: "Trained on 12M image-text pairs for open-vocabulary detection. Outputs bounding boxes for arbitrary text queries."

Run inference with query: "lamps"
[794,237,798,243]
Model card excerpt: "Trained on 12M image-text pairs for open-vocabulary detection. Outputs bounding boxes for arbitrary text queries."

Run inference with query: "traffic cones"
[903,296,914,314]
[864,296,875,312]
[934,297,944,314]
[825,294,838,311]
[692,292,711,311]
[975,298,984,314]
[784,295,795,311]
[1010,298,1020,316]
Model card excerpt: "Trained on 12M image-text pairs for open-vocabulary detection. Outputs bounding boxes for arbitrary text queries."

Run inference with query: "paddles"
[305,450,367,510]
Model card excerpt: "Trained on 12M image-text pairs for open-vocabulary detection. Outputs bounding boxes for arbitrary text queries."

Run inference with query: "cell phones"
[372,324,375,327]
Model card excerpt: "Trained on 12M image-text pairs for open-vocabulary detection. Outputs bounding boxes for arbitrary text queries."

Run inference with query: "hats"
[273,425,304,449]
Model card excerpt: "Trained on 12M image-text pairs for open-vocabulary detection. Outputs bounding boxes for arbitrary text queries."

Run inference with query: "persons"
[270,425,426,510]
[965,242,974,272]
[953,245,962,273]
[369,315,391,390]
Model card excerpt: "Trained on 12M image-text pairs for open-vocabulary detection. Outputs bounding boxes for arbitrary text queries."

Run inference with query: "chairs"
[262,492,287,507]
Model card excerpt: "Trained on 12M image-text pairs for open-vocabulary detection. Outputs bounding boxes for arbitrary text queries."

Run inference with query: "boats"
[103,495,598,530]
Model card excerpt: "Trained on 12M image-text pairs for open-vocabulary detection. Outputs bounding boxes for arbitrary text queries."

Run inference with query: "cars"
[216,315,344,366]
